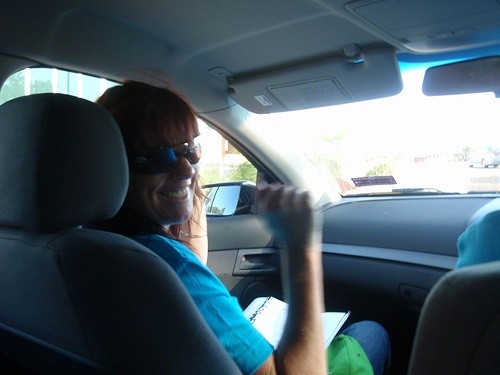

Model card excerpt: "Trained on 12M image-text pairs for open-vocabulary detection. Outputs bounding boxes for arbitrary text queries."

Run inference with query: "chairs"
[406,260,500,375]
[0,91,243,375]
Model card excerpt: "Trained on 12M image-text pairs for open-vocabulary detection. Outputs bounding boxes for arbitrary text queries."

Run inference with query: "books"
[242,297,350,363]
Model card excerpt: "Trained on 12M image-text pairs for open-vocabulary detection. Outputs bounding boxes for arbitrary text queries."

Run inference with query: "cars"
[469,146,500,169]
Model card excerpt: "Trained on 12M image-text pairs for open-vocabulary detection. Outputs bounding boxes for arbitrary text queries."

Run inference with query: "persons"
[455,199,500,269]
[84,79,394,375]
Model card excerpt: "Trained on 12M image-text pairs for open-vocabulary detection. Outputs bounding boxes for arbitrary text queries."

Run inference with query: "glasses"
[129,134,202,175]
